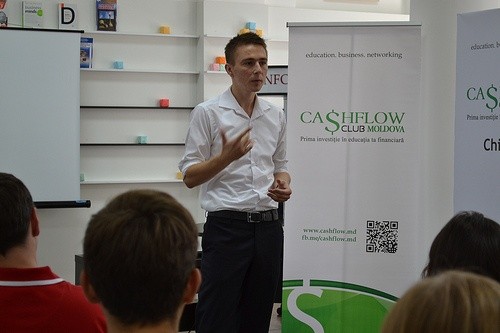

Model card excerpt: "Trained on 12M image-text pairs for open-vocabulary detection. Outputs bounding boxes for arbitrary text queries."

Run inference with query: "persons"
[178,32,292,333]
[421,210,500,283]
[380,269,500,333]
[0,173,109,333]
[81,190,202,333]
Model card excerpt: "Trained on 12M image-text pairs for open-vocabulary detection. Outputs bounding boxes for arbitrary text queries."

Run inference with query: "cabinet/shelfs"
[81,30,200,185]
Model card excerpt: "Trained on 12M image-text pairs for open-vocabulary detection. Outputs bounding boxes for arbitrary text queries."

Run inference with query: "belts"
[208,209,278,223]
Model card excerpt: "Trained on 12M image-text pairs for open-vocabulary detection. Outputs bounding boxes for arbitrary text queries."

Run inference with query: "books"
[0,0,117,69]
